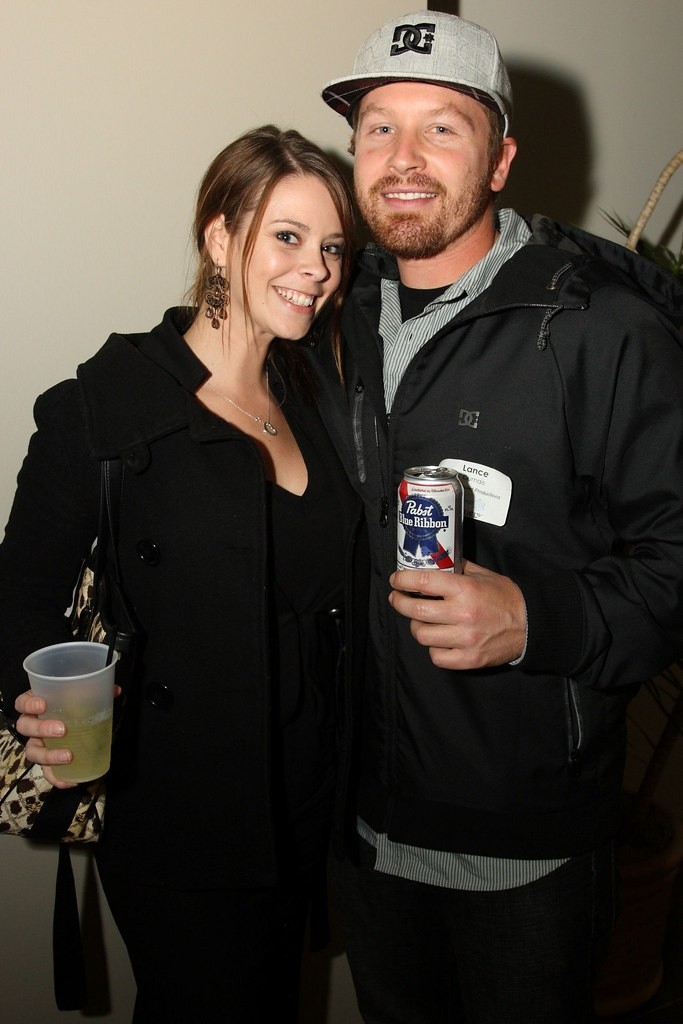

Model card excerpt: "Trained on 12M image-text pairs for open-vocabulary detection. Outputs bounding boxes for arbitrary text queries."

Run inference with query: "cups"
[23,642,118,784]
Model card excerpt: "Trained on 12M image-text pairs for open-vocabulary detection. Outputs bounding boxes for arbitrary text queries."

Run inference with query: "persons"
[0,126,363,1024]
[288,10,683,1024]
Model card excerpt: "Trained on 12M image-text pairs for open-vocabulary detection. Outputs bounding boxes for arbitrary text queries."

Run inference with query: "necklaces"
[206,363,278,436]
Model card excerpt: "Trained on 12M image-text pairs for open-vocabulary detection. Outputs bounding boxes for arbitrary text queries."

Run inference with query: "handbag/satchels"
[0,374,136,850]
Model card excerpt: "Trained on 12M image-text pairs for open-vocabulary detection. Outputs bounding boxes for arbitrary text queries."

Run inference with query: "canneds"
[396,465,464,595]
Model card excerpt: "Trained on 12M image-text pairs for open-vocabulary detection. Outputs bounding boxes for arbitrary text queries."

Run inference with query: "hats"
[321,11,514,139]
